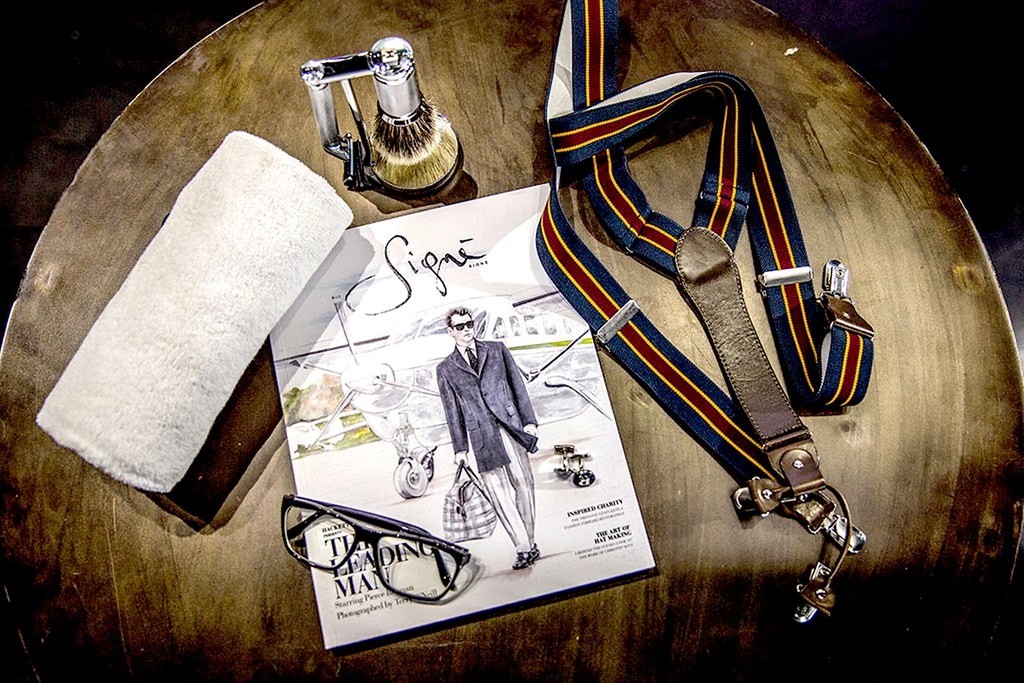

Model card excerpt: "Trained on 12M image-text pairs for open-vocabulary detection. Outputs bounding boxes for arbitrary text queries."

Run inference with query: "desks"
[0,0,1024,683]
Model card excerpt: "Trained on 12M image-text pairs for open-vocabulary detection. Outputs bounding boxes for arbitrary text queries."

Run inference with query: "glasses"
[281,494,471,603]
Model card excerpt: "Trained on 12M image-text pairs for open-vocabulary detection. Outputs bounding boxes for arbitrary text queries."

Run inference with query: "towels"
[36,130,356,494]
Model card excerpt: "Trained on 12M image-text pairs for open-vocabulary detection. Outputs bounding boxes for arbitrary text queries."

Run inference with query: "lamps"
[299,37,464,199]
